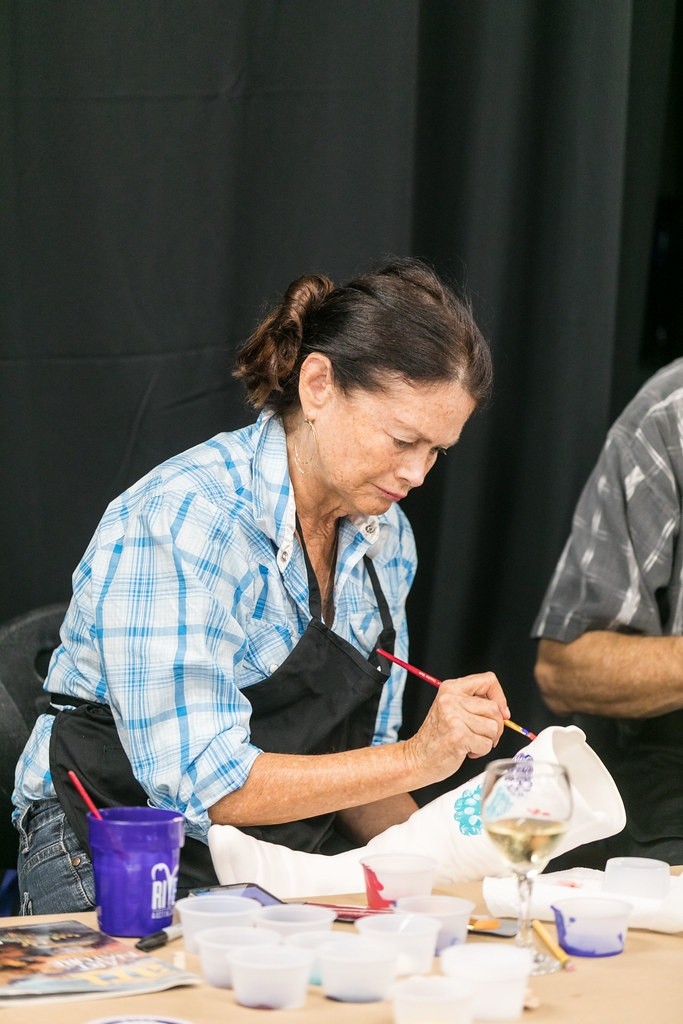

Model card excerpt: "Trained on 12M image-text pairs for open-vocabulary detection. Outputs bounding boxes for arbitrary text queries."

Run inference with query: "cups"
[87,806,185,937]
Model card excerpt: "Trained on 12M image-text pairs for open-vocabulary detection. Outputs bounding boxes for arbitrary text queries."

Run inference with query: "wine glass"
[479,757,573,976]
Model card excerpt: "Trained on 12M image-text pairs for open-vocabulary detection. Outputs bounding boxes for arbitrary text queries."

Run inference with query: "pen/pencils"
[531,918,576,974]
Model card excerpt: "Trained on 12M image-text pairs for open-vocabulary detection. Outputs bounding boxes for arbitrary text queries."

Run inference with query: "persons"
[529,358,683,877]
[11,257,512,914]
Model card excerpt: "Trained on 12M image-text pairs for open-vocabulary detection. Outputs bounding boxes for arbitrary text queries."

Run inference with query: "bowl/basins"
[551,898,636,956]
[355,913,442,976]
[603,856,670,905]
[176,894,262,953]
[315,938,403,1003]
[440,944,531,1019]
[360,853,442,909]
[294,929,359,968]
[390,895,475,958]
[203,927,277,988]
[388,975,472,1024]
[227,946,308,1007]
[256,903,337,936]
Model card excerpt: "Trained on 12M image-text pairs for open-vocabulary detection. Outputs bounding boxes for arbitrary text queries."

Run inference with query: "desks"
[1,869,683,1024]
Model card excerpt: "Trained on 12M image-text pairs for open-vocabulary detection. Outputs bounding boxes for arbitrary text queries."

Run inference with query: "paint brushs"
[375,647,538,742]
[68,769,131,865]
